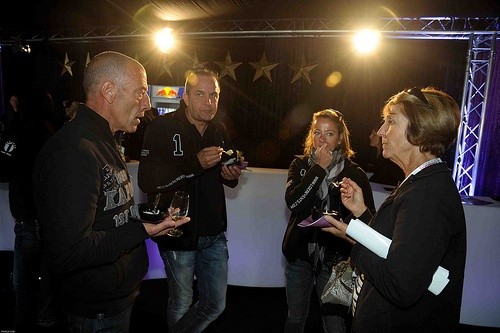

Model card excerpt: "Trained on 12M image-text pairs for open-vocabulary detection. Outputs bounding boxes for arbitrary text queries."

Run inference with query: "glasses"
[405,86,431,109]
[192,66,218,79]
[317,109,344,122]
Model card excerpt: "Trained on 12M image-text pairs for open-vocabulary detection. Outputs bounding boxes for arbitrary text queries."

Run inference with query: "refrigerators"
[148,85,184,116]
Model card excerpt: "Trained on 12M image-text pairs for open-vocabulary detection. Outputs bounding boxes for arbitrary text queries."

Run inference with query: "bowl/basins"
[138,202,162,222]
[222,163,237,167]
[312,208,341,222]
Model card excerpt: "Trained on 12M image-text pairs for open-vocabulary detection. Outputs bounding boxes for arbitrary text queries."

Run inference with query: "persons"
[34,51,191,333]
[281,108,377,333]
[367,126,406,186]
[321,88,467,333]
[3,95,22,130]
[137,69,241,333]
[8,91,57,333]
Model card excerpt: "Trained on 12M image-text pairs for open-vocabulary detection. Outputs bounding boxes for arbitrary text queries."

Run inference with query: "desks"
[123,160,499,328]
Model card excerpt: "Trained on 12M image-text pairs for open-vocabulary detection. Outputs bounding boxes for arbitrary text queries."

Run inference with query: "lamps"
[16,40,32,55]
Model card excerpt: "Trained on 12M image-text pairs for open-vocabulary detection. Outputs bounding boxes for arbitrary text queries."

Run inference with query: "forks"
[331,181,344,187]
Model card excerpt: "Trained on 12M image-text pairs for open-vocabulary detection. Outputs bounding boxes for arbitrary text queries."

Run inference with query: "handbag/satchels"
[320,256,355,310]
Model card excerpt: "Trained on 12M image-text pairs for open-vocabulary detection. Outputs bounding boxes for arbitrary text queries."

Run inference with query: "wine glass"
[166,191,189,237]
